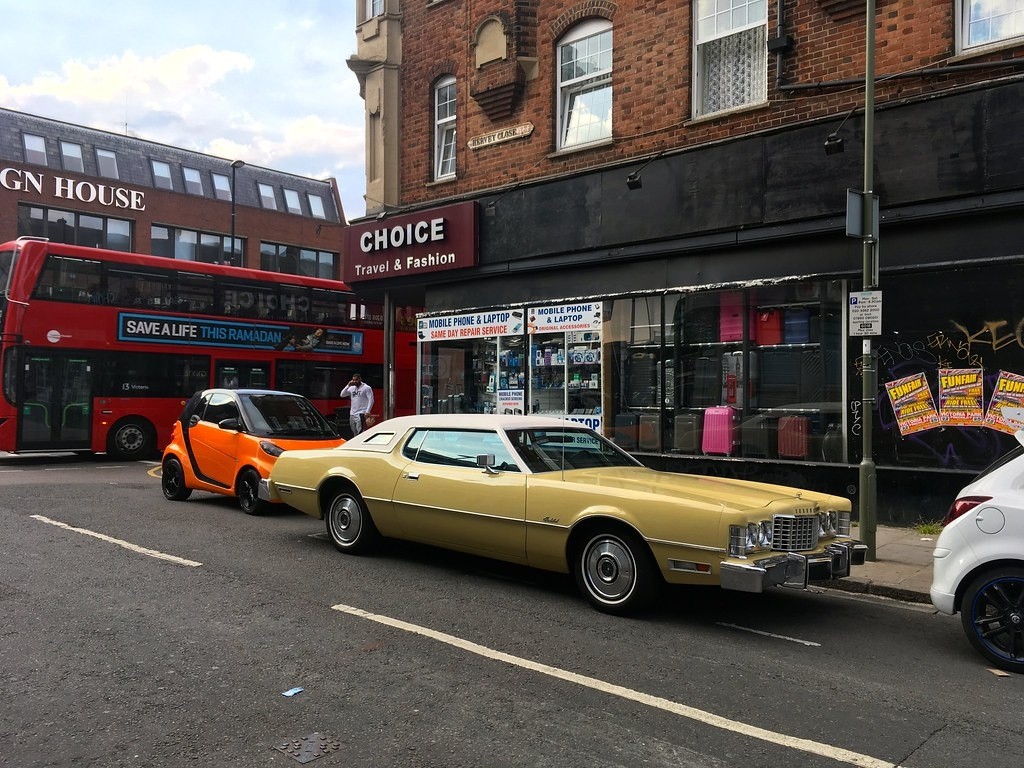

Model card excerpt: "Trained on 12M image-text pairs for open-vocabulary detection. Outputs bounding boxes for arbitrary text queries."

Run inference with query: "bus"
[0,234,424,458]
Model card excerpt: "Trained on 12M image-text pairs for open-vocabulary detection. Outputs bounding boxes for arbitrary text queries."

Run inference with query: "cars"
[268,413,870,616]
[928,430,1024,677]
[680,401,842,460]
[161,388,348,513]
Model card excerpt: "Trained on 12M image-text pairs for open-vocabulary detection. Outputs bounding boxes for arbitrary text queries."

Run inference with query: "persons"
[273,328,326,352]
[340,373,375,437]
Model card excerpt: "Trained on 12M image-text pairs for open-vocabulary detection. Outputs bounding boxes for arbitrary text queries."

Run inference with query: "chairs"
[42,281,372,329]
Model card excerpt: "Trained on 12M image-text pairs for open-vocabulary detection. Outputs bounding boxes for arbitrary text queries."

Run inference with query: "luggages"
[602,289,855,466]
[420,380,466,417]
